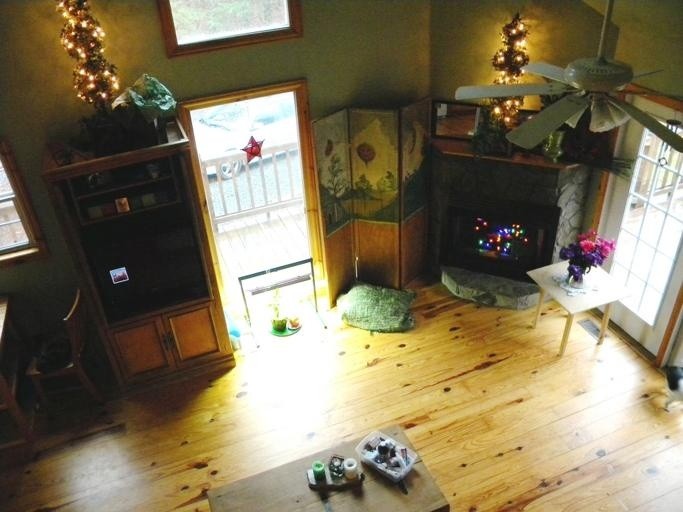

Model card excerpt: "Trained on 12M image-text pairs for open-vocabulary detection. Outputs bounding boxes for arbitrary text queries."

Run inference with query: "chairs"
[22,285,108,415]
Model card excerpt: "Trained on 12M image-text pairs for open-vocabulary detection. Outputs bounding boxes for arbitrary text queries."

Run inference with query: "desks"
[525,259,626,356]
[206,421,453,512]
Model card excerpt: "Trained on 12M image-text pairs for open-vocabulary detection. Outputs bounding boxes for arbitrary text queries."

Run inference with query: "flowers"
[558,232,616,271]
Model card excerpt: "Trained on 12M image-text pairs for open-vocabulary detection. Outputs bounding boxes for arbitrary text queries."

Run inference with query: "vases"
[564,268,587,289]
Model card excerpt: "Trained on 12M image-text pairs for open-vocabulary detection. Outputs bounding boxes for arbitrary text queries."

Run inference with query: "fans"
[454,0,682,152]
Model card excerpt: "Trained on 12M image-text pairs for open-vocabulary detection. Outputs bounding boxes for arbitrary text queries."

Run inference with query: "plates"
[270,322,301,336]
[287,322,301,330]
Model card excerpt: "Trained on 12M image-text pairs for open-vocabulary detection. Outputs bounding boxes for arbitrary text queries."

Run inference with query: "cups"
[343,459,358,480]
[327,454,346,477]
[272,316,287,331]
[311,461,325,480]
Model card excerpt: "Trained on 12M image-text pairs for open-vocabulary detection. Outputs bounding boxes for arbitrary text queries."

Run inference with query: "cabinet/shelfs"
[42,151,237,402]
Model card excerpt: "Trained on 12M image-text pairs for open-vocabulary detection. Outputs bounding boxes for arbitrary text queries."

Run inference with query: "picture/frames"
[156,0,304,56]
